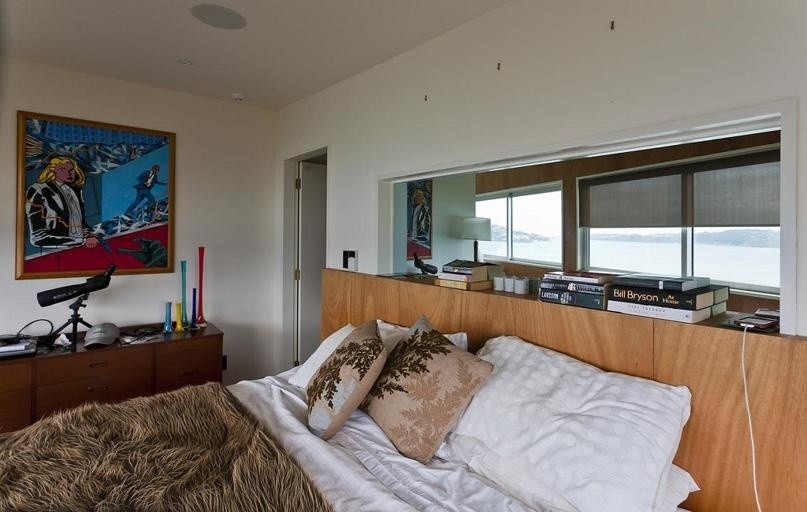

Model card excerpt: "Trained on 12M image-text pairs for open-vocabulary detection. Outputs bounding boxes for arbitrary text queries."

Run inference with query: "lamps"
[461,217,491,264]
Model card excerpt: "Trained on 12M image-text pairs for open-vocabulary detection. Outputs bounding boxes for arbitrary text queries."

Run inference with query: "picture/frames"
[14,110,175,280]
[407,178,433,260]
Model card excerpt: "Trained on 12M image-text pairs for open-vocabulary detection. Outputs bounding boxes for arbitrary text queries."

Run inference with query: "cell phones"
[734,315,778,328]
[755,307,780,318]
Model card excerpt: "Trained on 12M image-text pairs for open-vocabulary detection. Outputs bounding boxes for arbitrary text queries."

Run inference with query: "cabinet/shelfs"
[0,323,224,433]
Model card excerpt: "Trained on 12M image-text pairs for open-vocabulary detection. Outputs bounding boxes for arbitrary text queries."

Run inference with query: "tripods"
[37,312,93,352]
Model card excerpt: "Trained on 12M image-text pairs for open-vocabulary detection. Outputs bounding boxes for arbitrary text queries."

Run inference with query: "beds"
[0,361,700,512]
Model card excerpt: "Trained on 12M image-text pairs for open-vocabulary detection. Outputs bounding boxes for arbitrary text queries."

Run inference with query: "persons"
[125,164,169,216]
[423,181,432,210]
[24,157,100,250]
[410,188,430,243]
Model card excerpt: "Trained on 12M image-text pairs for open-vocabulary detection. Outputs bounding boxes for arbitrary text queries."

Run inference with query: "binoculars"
[414,252,438,274]
[37,264,116,307]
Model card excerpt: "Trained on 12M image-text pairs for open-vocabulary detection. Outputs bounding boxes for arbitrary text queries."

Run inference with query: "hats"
[84,323,119,347]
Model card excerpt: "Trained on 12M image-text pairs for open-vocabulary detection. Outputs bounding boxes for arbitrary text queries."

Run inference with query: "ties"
[147,173,154,179]
[63,189,82,235]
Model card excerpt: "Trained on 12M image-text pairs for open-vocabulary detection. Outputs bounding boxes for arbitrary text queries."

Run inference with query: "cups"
[491,274,531,295]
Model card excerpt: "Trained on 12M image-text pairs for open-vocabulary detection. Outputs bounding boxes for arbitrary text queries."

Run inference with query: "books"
[0,343,30,354]
[0,335,21,346]
[434,259,731,324]
